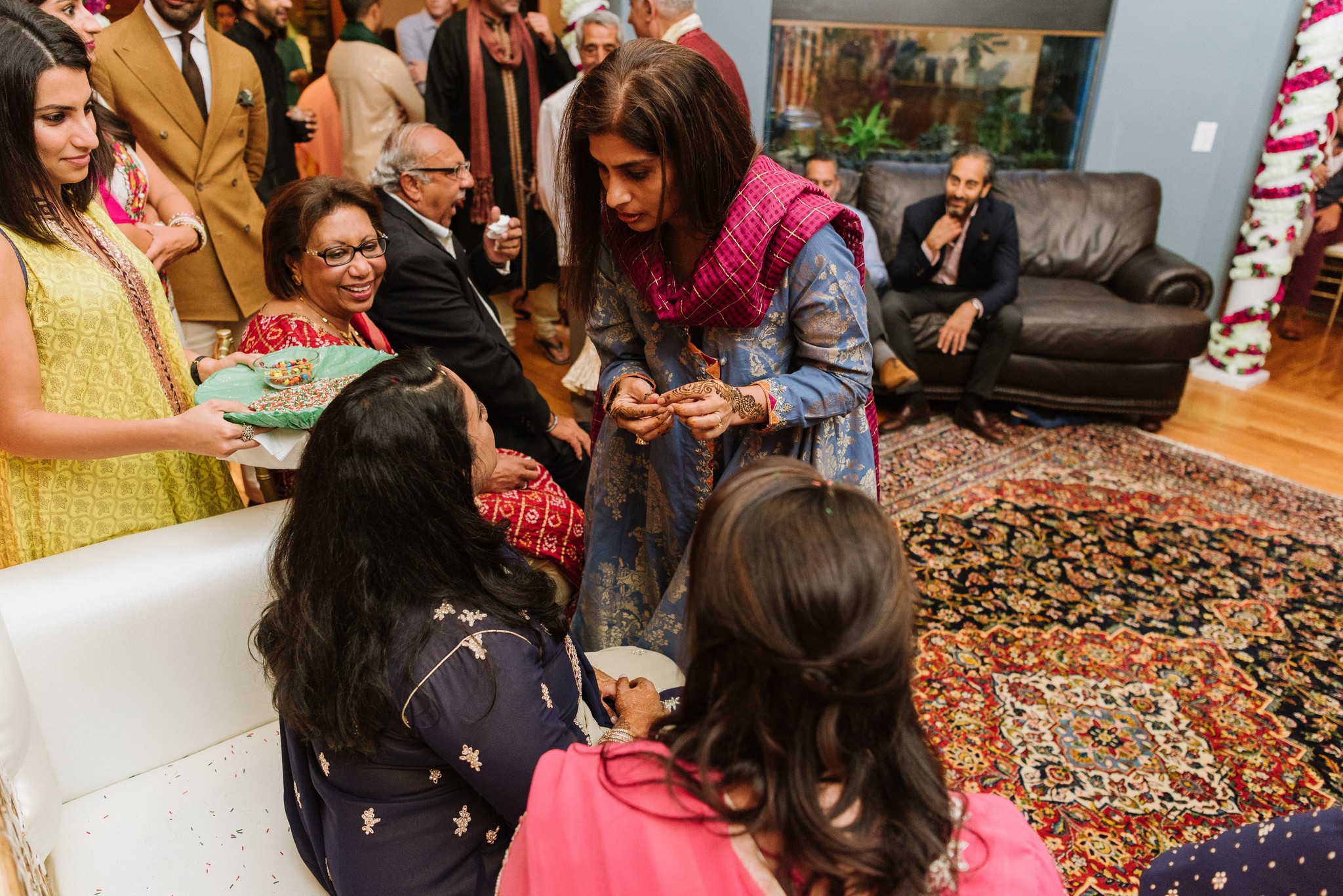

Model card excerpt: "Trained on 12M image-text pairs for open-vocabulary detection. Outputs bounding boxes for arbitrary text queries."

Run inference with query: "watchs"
[969,298,980,318]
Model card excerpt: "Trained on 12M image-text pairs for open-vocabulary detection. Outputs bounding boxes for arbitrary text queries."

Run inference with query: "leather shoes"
[955,403,1010,442]
[880,356,919,390]
[878,400,933,436]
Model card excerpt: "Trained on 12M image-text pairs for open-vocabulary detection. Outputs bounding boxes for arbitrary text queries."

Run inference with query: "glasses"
[300,227,388,265]
[407,159,471,179]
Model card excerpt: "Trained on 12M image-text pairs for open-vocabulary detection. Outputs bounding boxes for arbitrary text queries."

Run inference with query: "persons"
[326,0,430,206]
[625,0,752,138]
[796,151,923,396]
[206,0,343,205]
[876,140,1020,445]
[530,10,625,368]
[490,453,1072,896]
[240,172,591,592]
[359,122,594,509]
[0,0,265,572]
[242,348,689,895]
[393,0,468,126]
[555,38,881,675]
[83,0,275,357]
[1279,78,1343,340]
[18,0,207,358]
[423,0,577,296]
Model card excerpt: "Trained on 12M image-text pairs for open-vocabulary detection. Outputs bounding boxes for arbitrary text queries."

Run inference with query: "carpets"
[871,407,1343,896]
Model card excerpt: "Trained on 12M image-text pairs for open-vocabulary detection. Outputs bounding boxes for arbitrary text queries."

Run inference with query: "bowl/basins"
[251,349,320,389]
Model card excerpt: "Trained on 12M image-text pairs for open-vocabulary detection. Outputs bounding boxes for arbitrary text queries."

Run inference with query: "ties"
[174,33,209,126]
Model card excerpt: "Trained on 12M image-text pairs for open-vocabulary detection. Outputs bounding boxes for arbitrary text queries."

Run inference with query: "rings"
[520,480,528,491]
[635,433,650,446]
[713,412,724,429]
[241,422,254,443]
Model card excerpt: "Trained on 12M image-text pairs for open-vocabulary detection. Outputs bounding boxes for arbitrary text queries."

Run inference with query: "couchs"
[862,159,1215,434]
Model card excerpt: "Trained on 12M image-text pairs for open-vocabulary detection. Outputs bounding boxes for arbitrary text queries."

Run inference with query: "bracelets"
[166,211,208,255]
[545,409,558,432]
[297,290,369,349]
[1333,201,1343,211]
[188,356,211,384]
[598,727,640,744]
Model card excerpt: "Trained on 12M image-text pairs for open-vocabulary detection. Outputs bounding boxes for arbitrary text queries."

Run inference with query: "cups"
[289,102,316,144]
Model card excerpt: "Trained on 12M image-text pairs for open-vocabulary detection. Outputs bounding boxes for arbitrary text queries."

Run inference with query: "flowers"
[1207,1,1343,374]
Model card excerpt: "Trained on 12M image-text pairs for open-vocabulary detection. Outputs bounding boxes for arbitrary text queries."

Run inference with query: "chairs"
[1,497,332,896]
